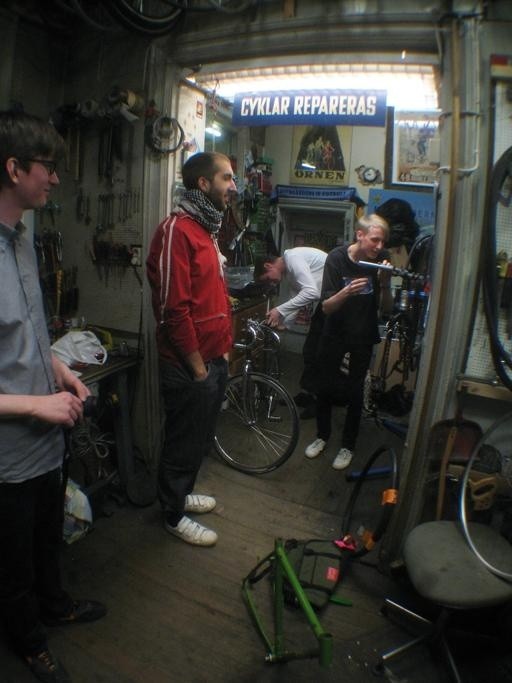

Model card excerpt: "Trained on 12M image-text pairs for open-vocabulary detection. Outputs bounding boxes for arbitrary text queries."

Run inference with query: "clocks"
[355,164,383,186]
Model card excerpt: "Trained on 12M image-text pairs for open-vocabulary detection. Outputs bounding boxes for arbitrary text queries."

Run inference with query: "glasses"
[21,155,57,177]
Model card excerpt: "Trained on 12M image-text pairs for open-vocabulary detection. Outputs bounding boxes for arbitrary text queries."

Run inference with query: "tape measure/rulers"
[65,98,100,118]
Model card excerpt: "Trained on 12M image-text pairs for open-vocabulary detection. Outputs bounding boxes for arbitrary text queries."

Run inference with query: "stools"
[383,517,508,677]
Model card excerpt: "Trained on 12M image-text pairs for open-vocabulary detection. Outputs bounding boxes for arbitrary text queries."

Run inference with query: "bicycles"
[214,315,300,476]
[362,232,432,428]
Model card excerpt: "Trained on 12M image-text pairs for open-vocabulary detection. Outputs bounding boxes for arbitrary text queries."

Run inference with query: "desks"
[50,344,143,501]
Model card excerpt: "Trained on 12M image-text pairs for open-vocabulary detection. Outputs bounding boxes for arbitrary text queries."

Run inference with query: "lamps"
[205,120,224,136]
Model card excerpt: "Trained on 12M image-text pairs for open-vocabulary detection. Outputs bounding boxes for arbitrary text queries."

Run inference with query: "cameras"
[78,393,104,418]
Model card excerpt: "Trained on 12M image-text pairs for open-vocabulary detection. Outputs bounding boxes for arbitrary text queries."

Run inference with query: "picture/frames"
[383,103,439,192]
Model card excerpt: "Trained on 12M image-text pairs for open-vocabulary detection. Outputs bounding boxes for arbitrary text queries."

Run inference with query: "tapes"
[107,86,145,122]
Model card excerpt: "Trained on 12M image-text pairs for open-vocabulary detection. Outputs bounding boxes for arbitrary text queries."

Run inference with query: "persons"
[254,246,328,419]
[145,151,236,546]
[0,112,108,680]
[305,135,336,170]
[304,213,392,471]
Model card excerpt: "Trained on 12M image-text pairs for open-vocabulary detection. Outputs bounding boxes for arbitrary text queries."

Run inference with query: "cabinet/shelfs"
[230,297,284,395]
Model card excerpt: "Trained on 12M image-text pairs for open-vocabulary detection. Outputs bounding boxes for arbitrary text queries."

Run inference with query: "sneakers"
[49,599,107,624]
[332,447,354,470]
[23,648,72,682]
[184,495,216,518]
[165,516,219,547]
[305,438,327,458]
[279,392,316,420]
